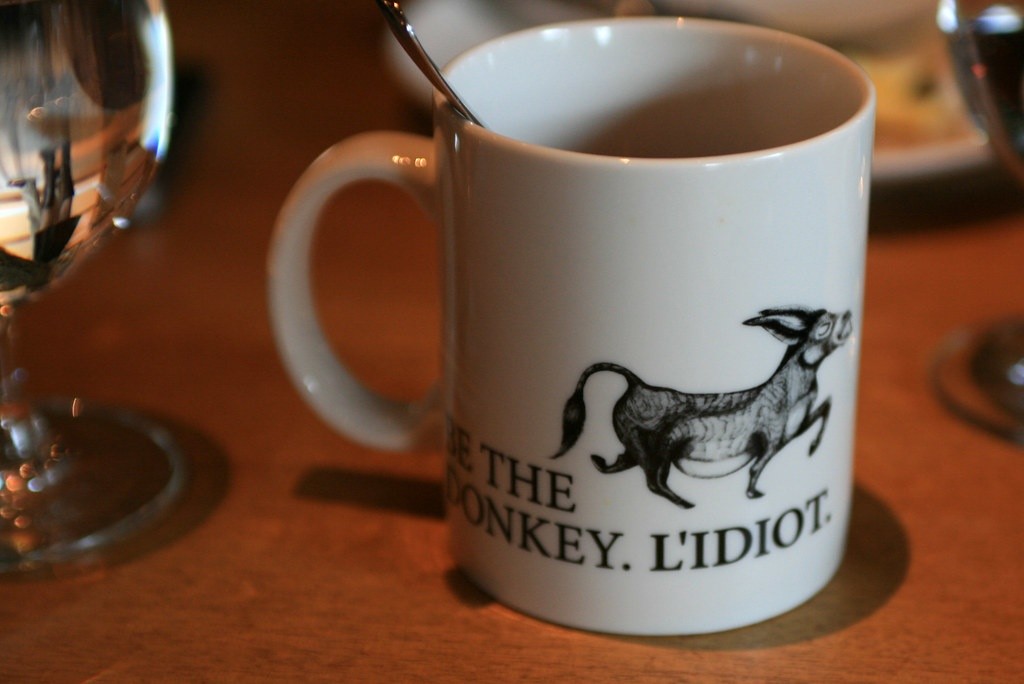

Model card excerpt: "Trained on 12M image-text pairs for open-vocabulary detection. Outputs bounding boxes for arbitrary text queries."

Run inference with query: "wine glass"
[925,0,1024,443]
[2,1,189,567]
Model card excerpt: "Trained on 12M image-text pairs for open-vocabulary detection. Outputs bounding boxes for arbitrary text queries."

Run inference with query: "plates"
[389,5,991,174]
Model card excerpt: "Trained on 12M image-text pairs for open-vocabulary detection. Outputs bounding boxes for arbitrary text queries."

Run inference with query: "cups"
[264,14,874,633]
